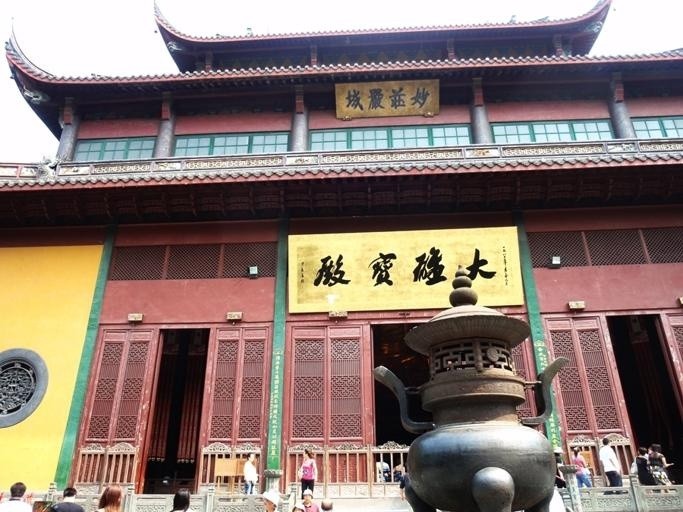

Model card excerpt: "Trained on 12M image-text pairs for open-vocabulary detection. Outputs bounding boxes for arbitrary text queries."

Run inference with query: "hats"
[261,488,279,507]
[302,489,314,499]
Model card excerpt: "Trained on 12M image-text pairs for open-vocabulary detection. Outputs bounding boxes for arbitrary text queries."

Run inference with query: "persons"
[572,447,592,497]
[297,449,317,500]
[169,488,195,512]
[399,473,409,500]
[50,487,85,512]
[0,482,32,512]
[552,447,566,488]
[244,452,258,494]
[636,444,674,493]
[94,484,123,512]
[599,438,623,494]
[262,489,333,512]
